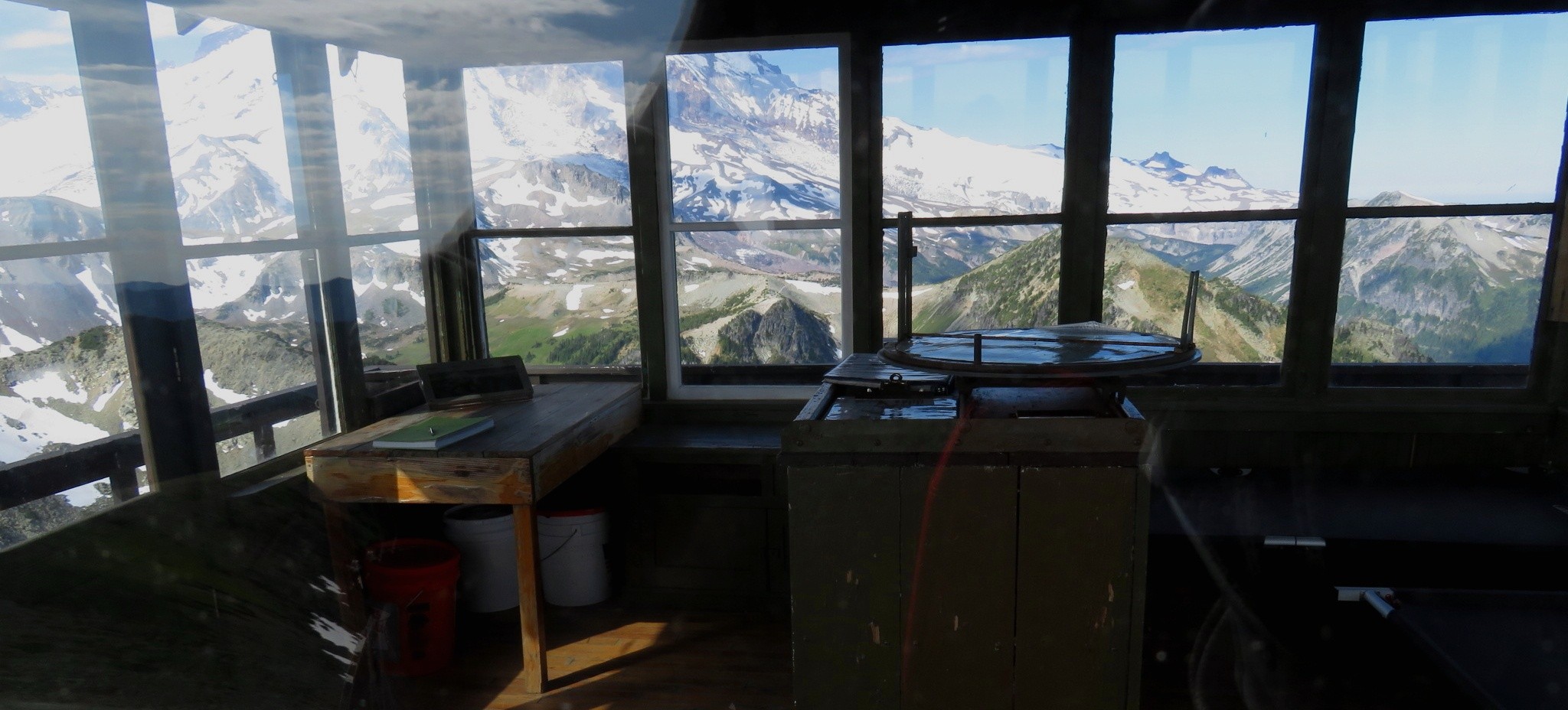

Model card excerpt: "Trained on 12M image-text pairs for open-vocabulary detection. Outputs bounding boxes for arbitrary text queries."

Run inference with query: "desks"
[1332,585,1568,709]
[304,380,642,694]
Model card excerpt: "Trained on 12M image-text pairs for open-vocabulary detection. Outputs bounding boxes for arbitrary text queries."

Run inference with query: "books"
[372,414,496,451]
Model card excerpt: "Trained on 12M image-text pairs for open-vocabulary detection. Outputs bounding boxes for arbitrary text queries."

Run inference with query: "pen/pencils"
[429,426,434,435]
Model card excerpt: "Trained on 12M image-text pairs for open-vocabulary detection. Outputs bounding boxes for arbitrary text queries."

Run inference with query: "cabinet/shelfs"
[787,382,1167,709]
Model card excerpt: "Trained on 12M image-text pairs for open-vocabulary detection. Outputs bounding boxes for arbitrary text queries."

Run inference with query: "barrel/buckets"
[439,504,539,613]
[539,511,611,608]
[362,538,459,680]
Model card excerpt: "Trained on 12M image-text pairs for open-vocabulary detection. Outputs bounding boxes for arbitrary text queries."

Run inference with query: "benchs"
[614,419,785,615]
[1152,467,1568,577]
